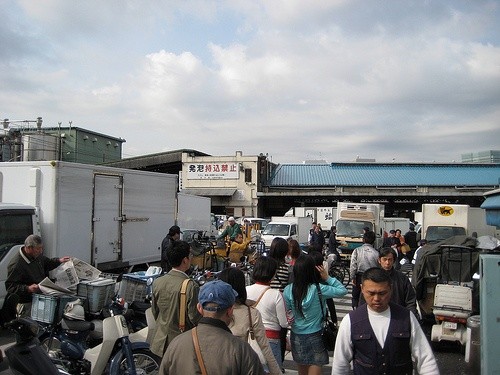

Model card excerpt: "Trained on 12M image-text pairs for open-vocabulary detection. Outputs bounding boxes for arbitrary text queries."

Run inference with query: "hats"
[169,226,183,235]
[197,280,239,311]
[389,230,396,234]
[228,216,235,221]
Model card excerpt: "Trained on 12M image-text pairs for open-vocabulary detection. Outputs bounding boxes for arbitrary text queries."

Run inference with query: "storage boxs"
[75,273,121,315]
[31,295,81,325]
[121,268,165,301]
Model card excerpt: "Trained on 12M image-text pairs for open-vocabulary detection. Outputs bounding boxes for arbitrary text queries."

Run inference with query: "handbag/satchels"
[401,242,411,253]
[324,320,337,351]
[393,247,398,257]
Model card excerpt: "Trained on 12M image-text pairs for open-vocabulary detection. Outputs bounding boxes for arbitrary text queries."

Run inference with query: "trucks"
[173,186,499,349]
[0,160,178,277]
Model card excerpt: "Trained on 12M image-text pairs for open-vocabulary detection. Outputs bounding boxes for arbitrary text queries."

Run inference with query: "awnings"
[179,189,237,196]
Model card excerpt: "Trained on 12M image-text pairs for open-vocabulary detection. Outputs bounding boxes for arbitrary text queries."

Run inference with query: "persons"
[411,239,428,271]
[329,226,341,262]
[331,266,442,375]
[218,267,284,375]
[231,234,250,252]
[217,216,243,244]
[160,224,183,273]
[268,237,294,374]
[311,226,325,254]
[308,222,317,244]
[284,254,348,375]
[363,226,370,233]
[405,226,418,265]
[381,229,401,258]
[285,239,305,265]
[245,256,296,375]
[159,280,265,375]
[349,231,380,310]
[359,247,420,320]
[151,240,200,359]
[4,235,71,304]
[395,229,407,269]
[307,252,340,328]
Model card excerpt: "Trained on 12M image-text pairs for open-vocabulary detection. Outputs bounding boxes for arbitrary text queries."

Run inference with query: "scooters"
[7,293,162,375]
